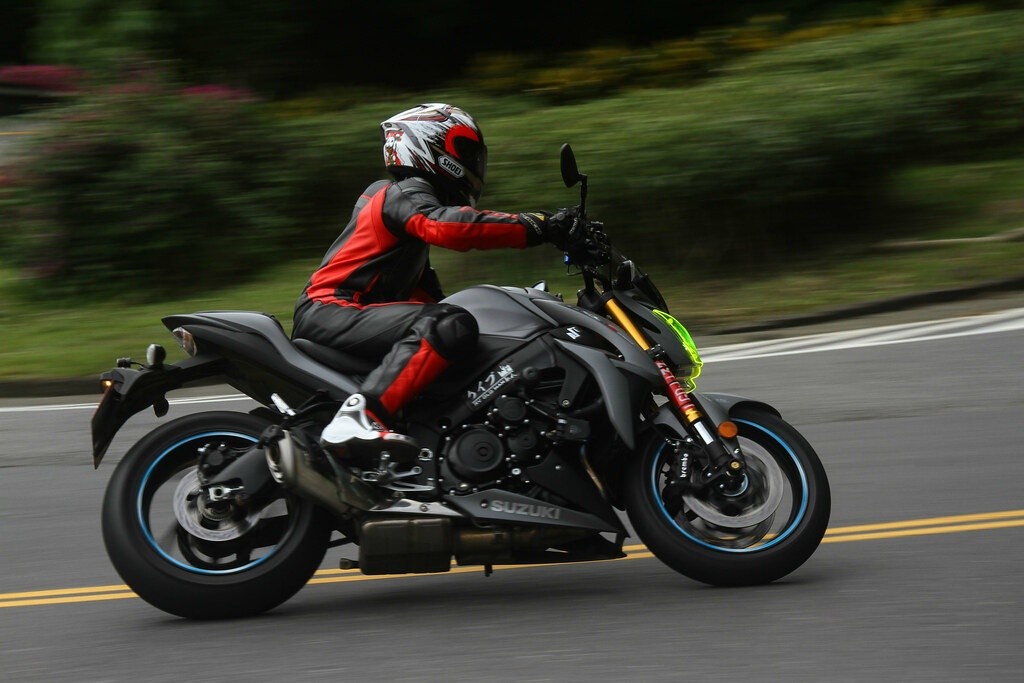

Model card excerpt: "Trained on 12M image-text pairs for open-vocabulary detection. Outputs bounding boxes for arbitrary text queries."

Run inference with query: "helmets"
[380,102,484,207]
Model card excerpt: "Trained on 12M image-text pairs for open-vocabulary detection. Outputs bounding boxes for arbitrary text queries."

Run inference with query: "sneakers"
[320,392,420,462]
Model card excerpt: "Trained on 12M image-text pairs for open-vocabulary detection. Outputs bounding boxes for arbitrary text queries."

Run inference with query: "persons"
[290,101,571,455]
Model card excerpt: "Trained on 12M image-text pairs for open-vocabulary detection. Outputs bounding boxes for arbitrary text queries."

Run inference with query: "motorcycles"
[91,142,831,621]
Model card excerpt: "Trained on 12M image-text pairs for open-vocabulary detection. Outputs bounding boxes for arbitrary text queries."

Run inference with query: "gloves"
[546,210,586,250]
